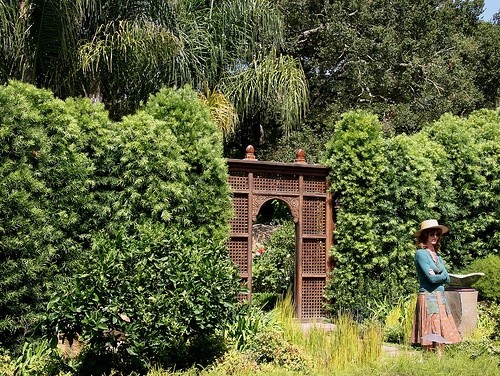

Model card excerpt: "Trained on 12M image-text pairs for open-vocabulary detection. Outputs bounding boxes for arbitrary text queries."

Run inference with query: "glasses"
[430,233,440,237]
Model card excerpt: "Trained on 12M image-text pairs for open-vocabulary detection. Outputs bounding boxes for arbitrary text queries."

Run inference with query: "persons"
[410,219,462,358]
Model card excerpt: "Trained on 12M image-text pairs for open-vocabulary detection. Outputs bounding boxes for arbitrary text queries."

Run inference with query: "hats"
[413,219,449,238]
